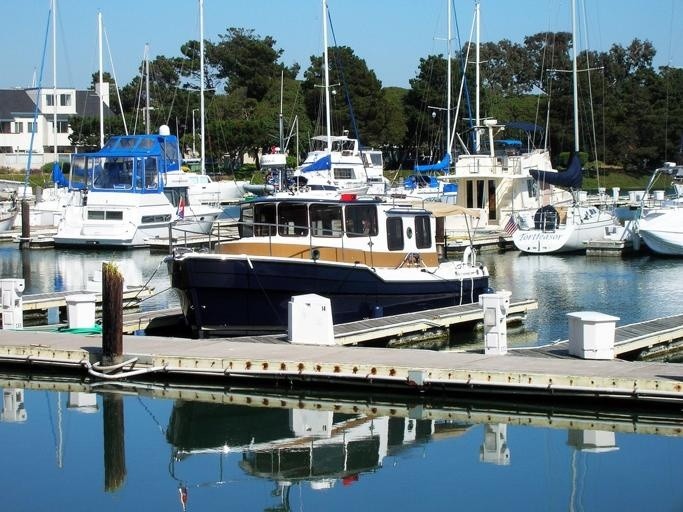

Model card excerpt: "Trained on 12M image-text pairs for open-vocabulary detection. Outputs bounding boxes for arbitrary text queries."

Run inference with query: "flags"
[176,196,185,219]
[504,216,518,237]
[301,154,331,173]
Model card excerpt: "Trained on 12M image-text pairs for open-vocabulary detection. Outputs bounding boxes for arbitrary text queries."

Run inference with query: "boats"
[165,186,492,338]
[165,403,477,511]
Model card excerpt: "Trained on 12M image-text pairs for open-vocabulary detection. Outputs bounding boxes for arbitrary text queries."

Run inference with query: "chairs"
[443,184,457,204]
